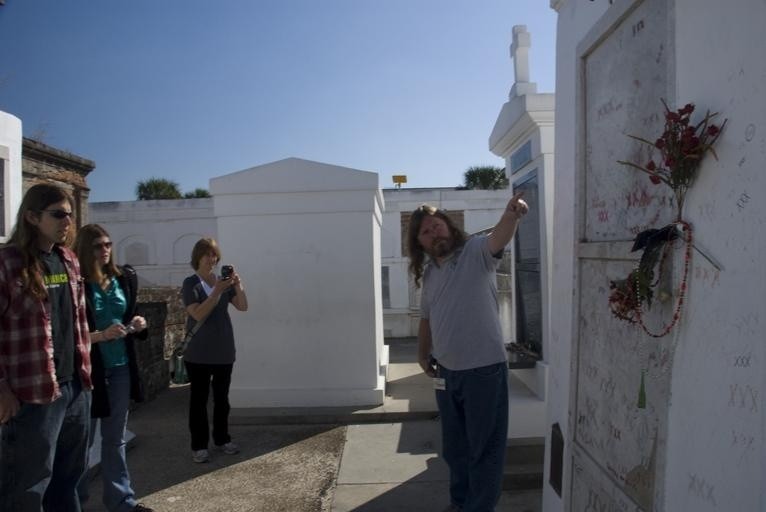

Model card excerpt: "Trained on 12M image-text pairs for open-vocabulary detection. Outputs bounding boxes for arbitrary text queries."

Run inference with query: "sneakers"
[192,449,209,463]
[211,440,240,455]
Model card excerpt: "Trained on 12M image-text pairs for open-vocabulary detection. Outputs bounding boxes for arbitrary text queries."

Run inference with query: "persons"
[181,236,251,464]
[0,180,96,512]
[406,189,531,511]
[69,225,153,510]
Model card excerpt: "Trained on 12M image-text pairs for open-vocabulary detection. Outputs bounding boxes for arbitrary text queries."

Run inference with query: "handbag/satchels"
[169,339,190,384]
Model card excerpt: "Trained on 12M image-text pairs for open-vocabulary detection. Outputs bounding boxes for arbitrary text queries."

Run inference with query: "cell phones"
[222,266,233,281]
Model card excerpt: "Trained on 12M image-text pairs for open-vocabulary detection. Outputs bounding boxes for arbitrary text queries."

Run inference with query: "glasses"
[92,241,112,250]
[35,209,73,219]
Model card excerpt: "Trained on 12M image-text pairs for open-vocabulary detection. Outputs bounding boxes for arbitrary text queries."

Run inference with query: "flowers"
[611,96,730,225]
[595,228,693,326]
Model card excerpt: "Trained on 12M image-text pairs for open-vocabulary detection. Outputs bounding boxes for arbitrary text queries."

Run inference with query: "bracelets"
[102,328,109,341]
[230,277,242,288]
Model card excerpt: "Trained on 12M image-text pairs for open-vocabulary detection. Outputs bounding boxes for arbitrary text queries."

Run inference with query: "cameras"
[122,321,137,333]
